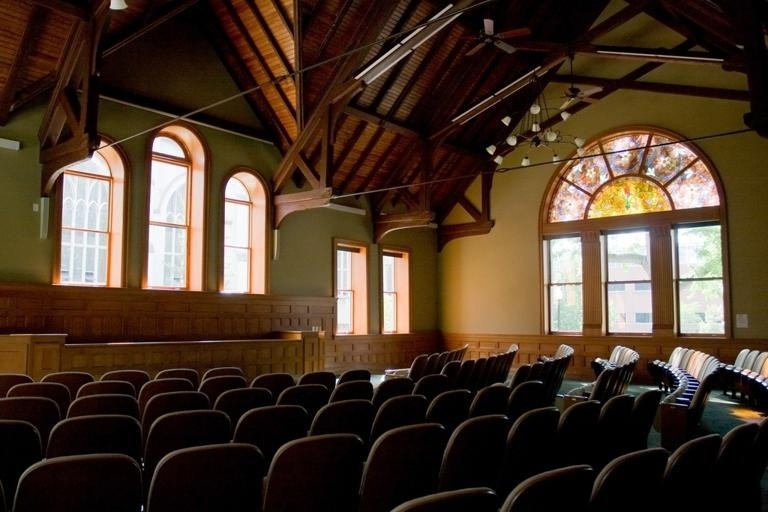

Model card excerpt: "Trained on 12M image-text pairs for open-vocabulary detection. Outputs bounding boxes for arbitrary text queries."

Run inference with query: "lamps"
[483,69,587,171]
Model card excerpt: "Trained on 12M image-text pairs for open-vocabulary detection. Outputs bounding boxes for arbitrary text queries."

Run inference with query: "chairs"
[1,338,768,512]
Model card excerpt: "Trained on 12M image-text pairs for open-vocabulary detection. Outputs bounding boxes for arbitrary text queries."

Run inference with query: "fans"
[560,50,601,112]
[454,1,530,57]
